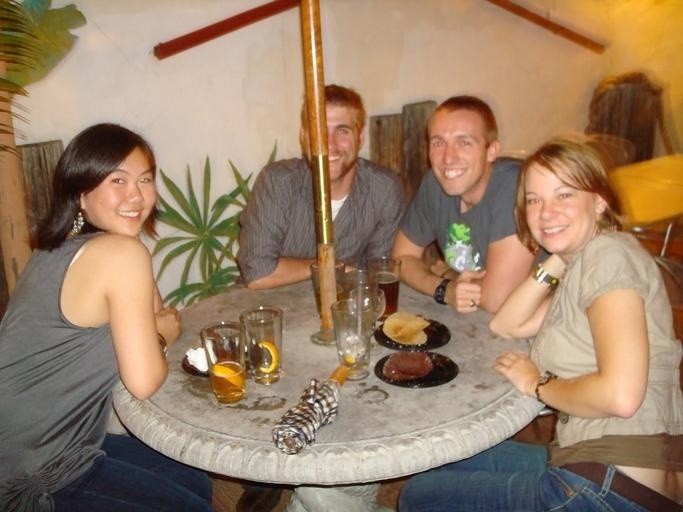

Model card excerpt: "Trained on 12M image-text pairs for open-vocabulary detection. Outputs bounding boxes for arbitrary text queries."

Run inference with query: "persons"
[390,94,536,317]
[0,123,220,512]
[393,139,683,511]
[232,85,409,290]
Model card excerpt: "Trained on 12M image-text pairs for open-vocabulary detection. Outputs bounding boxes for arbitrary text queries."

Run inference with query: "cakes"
[179,333,238,371]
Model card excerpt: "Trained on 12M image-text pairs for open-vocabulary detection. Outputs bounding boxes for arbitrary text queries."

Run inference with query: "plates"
[375,353,457,387]
[374,317,451,349]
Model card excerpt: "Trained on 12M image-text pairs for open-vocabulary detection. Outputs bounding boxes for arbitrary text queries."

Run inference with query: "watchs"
[535,371,555,401]
[433,277,450,305]
[156,330,167,353]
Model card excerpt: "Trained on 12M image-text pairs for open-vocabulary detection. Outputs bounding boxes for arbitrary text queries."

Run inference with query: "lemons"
[211,363,246,386]
[257,342,280,373]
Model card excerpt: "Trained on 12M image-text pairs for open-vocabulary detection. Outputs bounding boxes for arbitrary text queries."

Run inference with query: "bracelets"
[531,263,557,290]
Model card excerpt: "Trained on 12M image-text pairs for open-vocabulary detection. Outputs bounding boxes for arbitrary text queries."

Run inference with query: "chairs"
[577,70,674,164]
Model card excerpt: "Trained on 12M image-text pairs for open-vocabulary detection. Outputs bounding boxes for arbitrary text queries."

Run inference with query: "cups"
[309,255,401,382]
[239,303,282,383]
[202,319,245,403]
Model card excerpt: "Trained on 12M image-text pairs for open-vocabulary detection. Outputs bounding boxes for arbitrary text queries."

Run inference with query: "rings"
[469,299,475,307]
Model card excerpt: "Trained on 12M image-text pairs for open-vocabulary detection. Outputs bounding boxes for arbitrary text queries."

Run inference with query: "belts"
[565,461,683,512]
